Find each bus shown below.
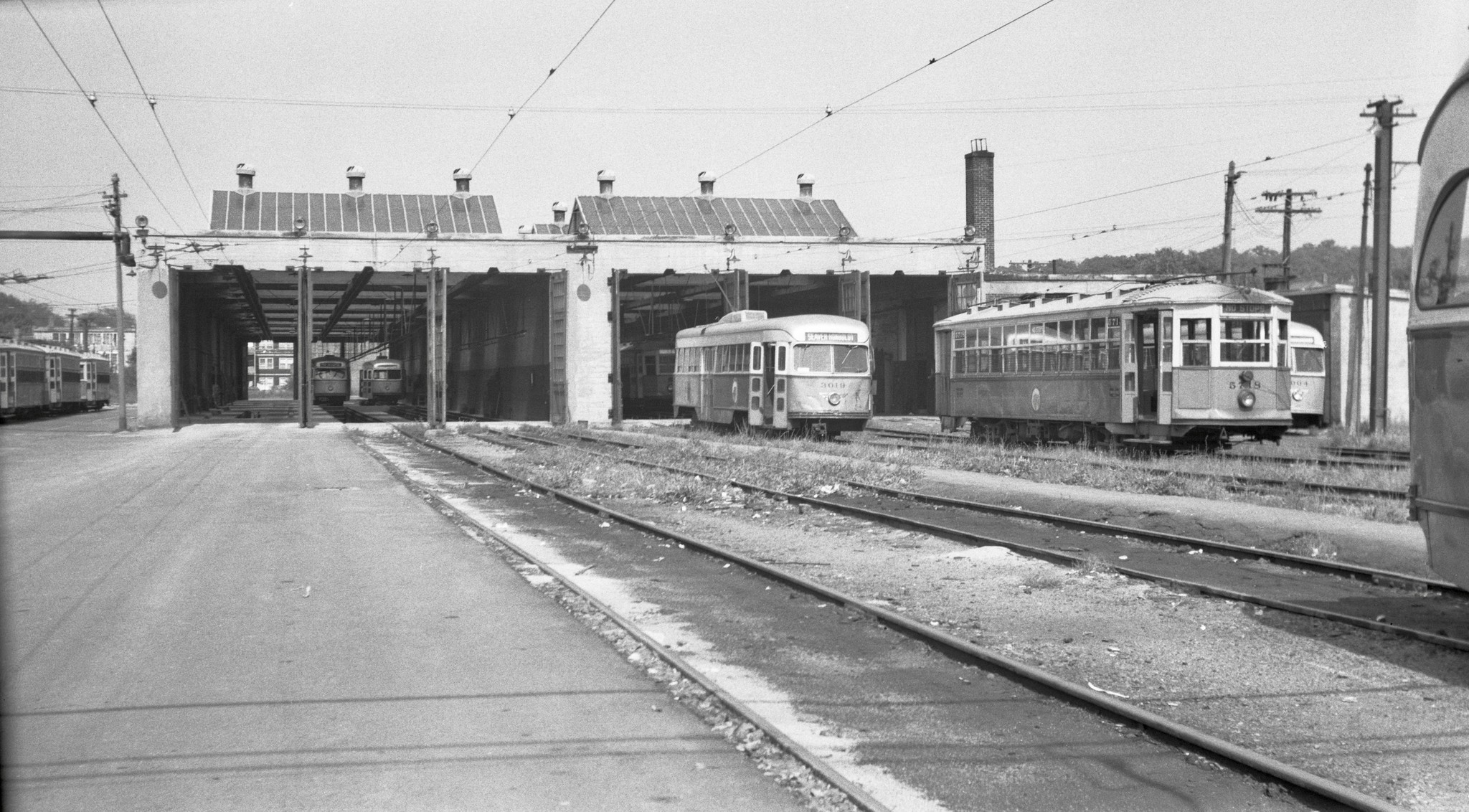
[621,332,676,412]
[1404,55,1468,596]
[931,266,1293,455]
[1291,321,1326,424]
[359,354,403,402]
[672,264,876,443]
[0,343,109,419]
[311,354,350,405]
